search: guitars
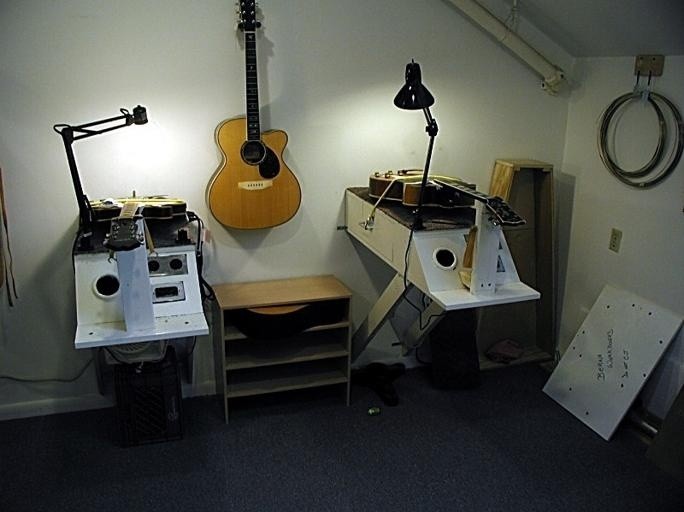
[369,169,526,227]
[87,194,187,250]
[206,0,301,230]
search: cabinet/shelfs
[211,274,352,425]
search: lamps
[394,59,439,230]
[62,105,148,235]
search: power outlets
[610,228,621,254]
